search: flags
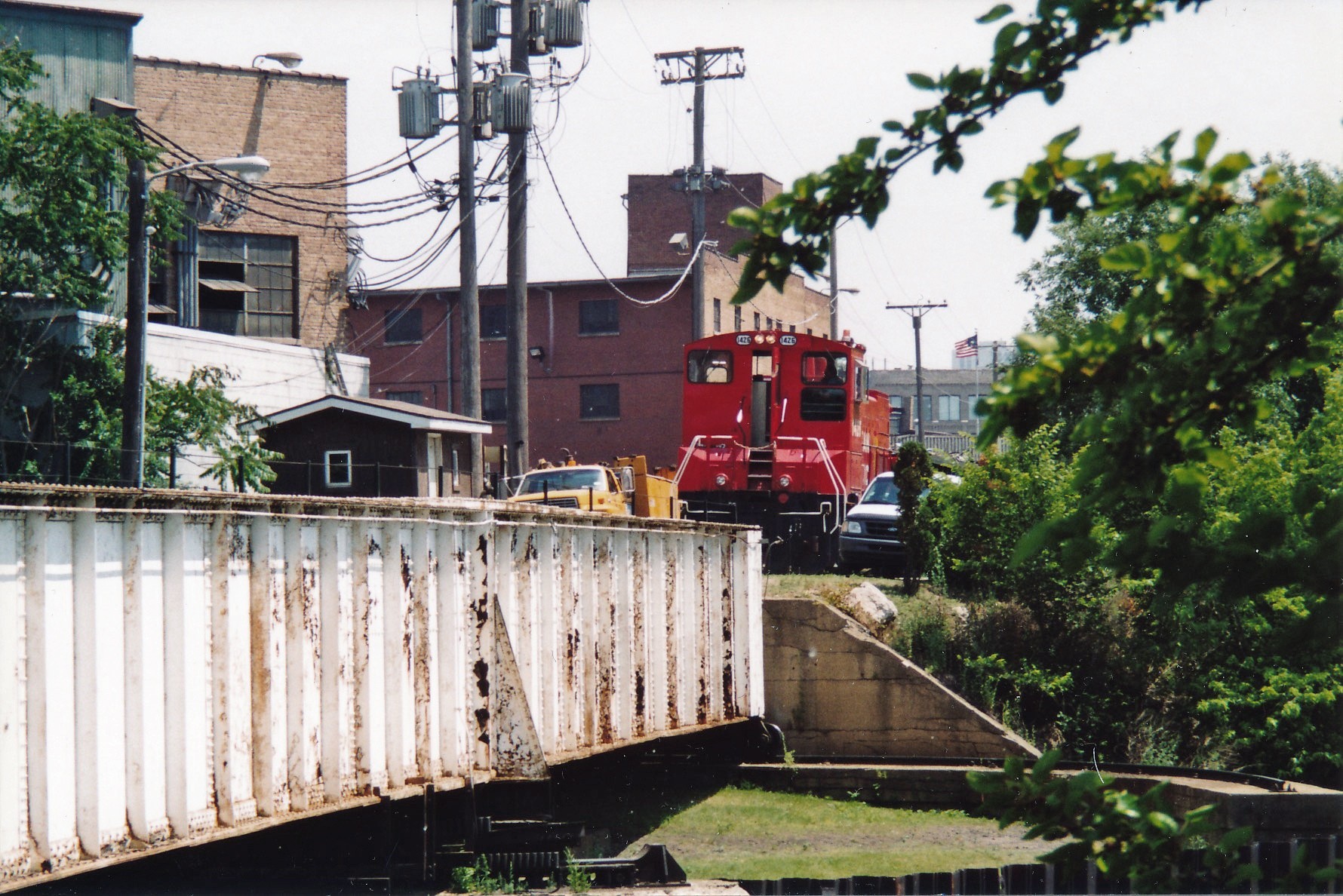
[955,334,977,357]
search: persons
[820,365,843,383]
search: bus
[674,325,900,566]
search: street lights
[120,147,269,491]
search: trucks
[507,447,682,519]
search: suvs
[839,471,967,571]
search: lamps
[528,346,544,360]
[253,51,303,73]
